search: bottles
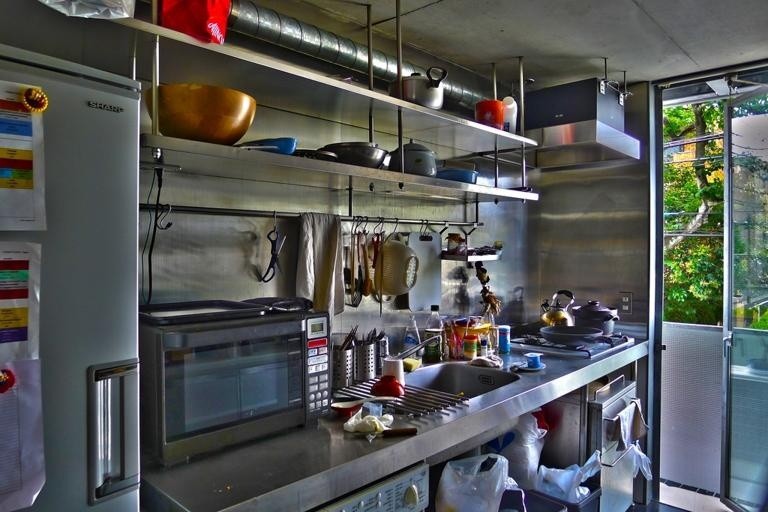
[501,97,518,134]
[480,340,487,355]
[498,325,511,354]
[446,232,466,256]
[424,304,445,358]
[482,311,495,328]
[402,315,421,358]
[462,335,478,361]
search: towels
[288,212,346,337]
[616,398,650,451]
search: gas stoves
[510,332,635,359]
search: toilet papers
[381,358,405,386]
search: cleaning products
[422,304,446,364]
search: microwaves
[145,309,332,468]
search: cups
[377,340,388,374]
[523,353,544,368]
[382,357,406,395]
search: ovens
[588,372,636,512]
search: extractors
[524,76,640,175]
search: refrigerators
[1,43,144,512]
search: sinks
[403,361,521,400]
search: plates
[514,362,546,370]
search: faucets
[390,336,446,361]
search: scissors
[259,230,288,283]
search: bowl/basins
[447,322,492,358]
[369,375,405,396]
[317,141,388,167]
[147,84,257,148]
[437,169,478,184]
[244,137,297,155]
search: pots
[540,325,612,348]
[572,299,618,335]
[389,65,448,110]
[387,138,437,178]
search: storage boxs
[532,469,602,512]
[525,491,568,512]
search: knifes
[353,427,417,438]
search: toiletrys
[401,315,423,365]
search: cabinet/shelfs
[0,0,539,204]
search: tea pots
[539,289,575,327]
